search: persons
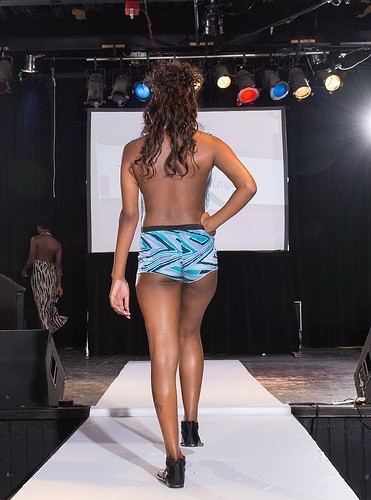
[20,216,70,334]
[106,62,259,489]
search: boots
[181,420,201,447]
[156,455,186,488]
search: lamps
[289,67,311,100]
[261,69,290,101]
[132,77,154,103]
[110,75,129,108]
[0,51,14,95]
[314,63,341,92]
[233,69,260,104]
[21,53,47,74]
[86,72,105,108]
[204,12,225,35]
[214,64,232,89]
[0,328,67,408]
[353,330,370,406]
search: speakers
[354,328,371,405]
[0,330,65,408]
